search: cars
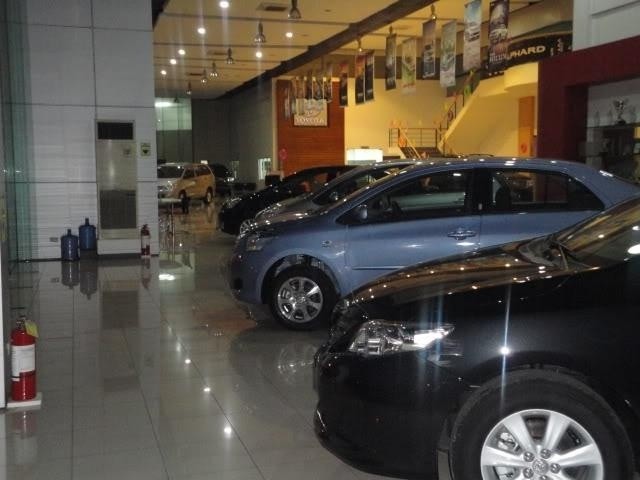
[402,19,508,76]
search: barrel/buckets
[60,262,80,290]
[257,157,272,181]
[60,229,80,262]
[80,253,99,301]
[79,217,98,251]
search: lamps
[172,0,301,103]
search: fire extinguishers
[10,315,39,401]
[140,223,151,256]
